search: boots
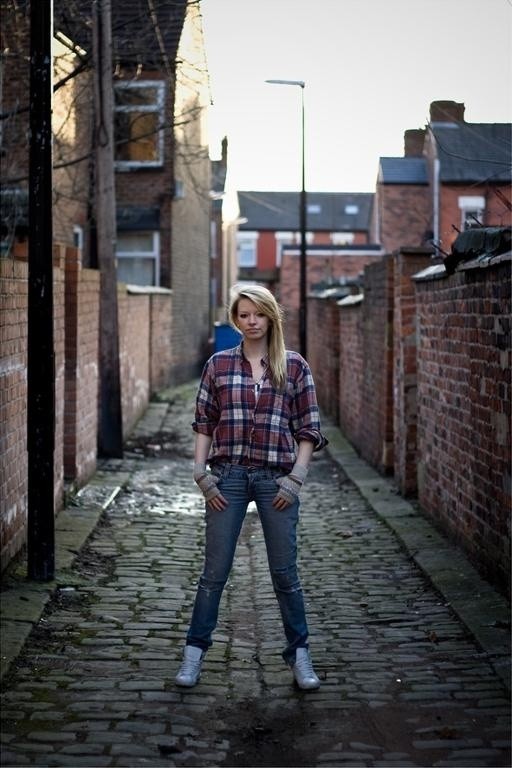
[174,646,205,687]
[293,648,321,691]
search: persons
[176,286,329,690]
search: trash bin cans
[214,321,243,353]
